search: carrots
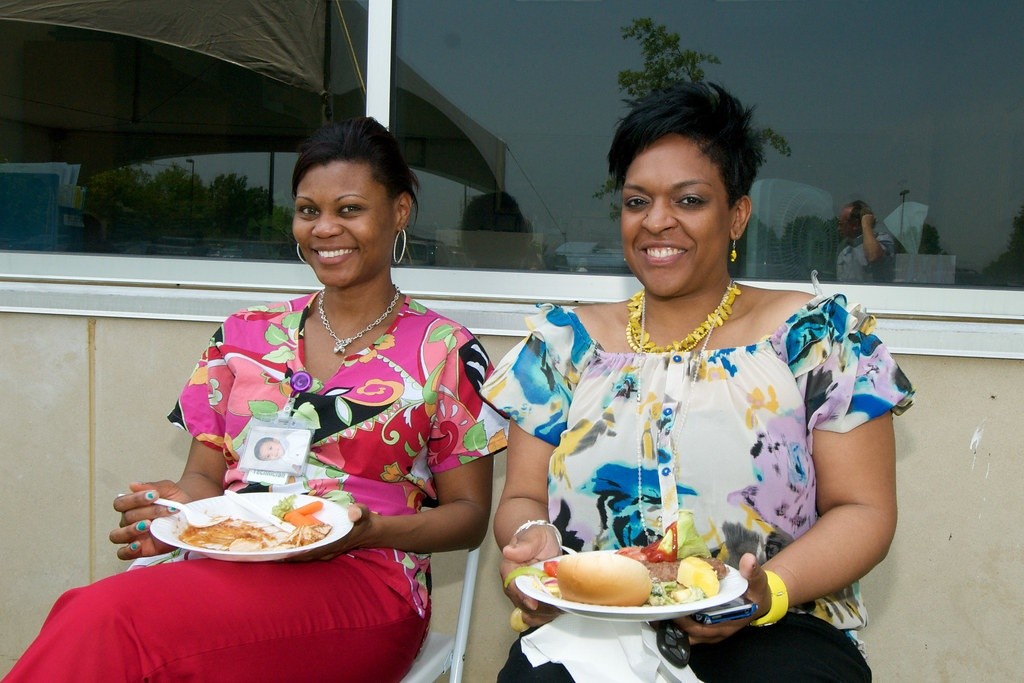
[283,501,323,528]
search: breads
[556,551,652,607]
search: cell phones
[690,596,758,626]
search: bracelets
[749,570,789,627]
[512,520,578,555]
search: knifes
[224,490,296,533]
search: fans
[745,178,841,281]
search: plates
[515,549,748,622]
[150,492,354,561]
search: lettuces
[653,509,712,560]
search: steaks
[636,555,725,582]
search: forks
[117,493,229,528]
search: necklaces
[314,286,401,356]
[626,280,742,542]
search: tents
[0,0,507,261]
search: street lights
[186,157,196,240]
[896,188,913,249]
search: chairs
[125,544,481,683]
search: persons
[0,117,507,683]
[481,81,916,683]
[835,201,897,284]
